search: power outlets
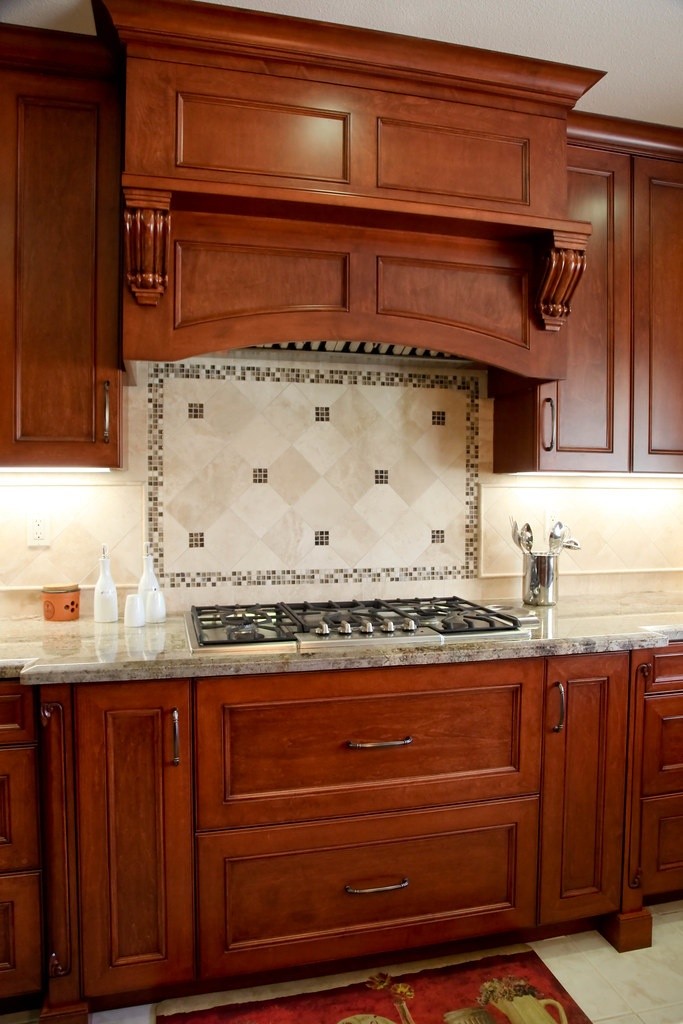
[28,513,49,547]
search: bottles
[138,542,160,597]
[93,543,118,622]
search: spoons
[549,521,564,555]
[520,523,533,554]
[512,521,525,554]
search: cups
[123,594,145,627]
[145,591,166,623]
[522,552,558,606]
[41,584,81,621]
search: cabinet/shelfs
[88,1,606,378]
[39,678,192,1024]
[196,652,547,982]
[540,646,631,959]
[0,681,37,1009]
[494,111,681,472]
[633,644,683,952]
[0,24,121,469]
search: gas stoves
[184,595,532,658]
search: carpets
[152,941,594,1024]
